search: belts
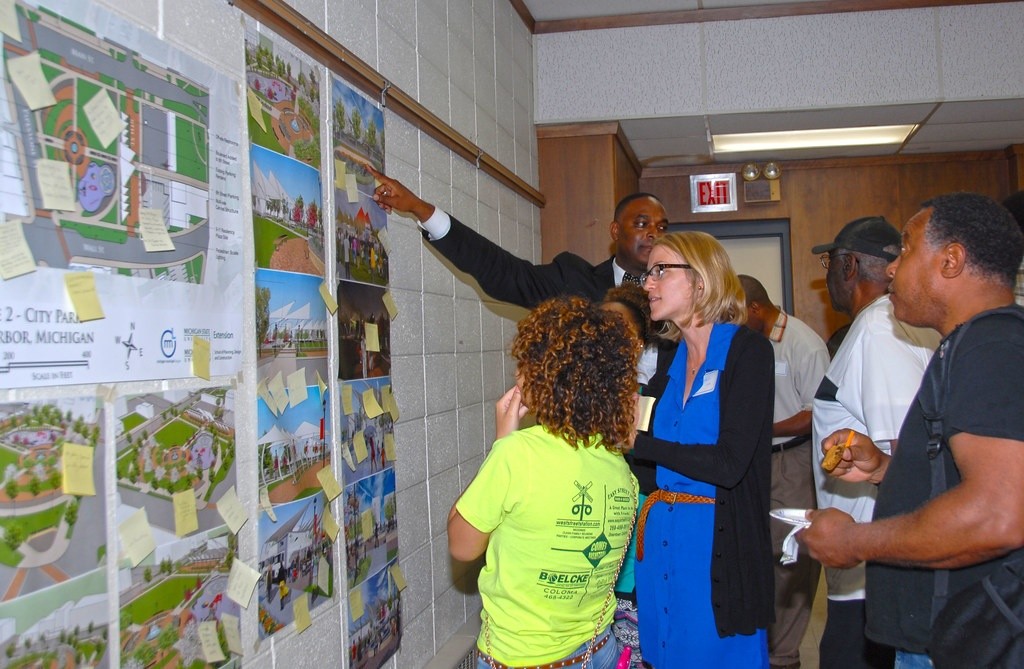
[636,489,716,561]
[771,432,812,453]
[474,633,612,669]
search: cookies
[822,446,843,471]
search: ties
[622,272,642,287]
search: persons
[365,163,671,310]
[737,274,831,669]
[447,285,663,668]
[274,576,288,610]
[369,436,386,474]
[342,242,389,283]
[811,214,943,669]
[624,231,775,669]
[348,635,363,669]
[374,522,379,548]
[795,193,1024,669]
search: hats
[810,215,903,263]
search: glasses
[638,263,692,284]
[819,251,861,269]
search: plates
[769,508,812,528]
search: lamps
[740,160,781,203]
